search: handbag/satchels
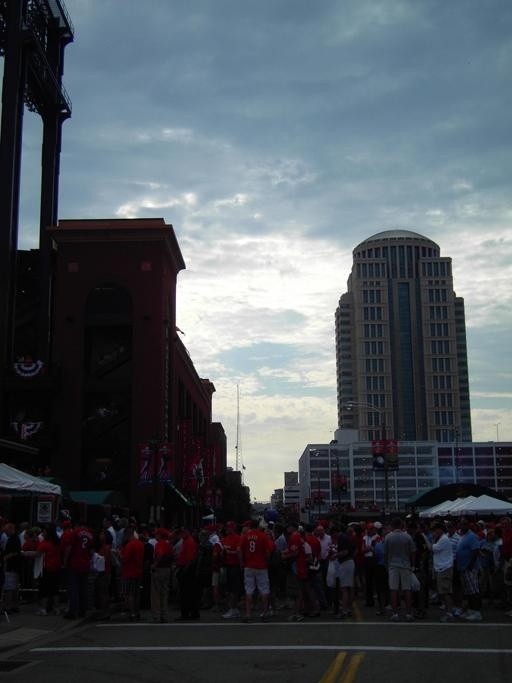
[267,551,284,576]
[158,555,172,568]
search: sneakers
[221,604,483,625]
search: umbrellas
[419,492,511,516]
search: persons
[1,512,512,626]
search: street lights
[342,397,393,514]
[327,439,343,506]
[308,448,322,518]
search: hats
[0,516,512,537]
